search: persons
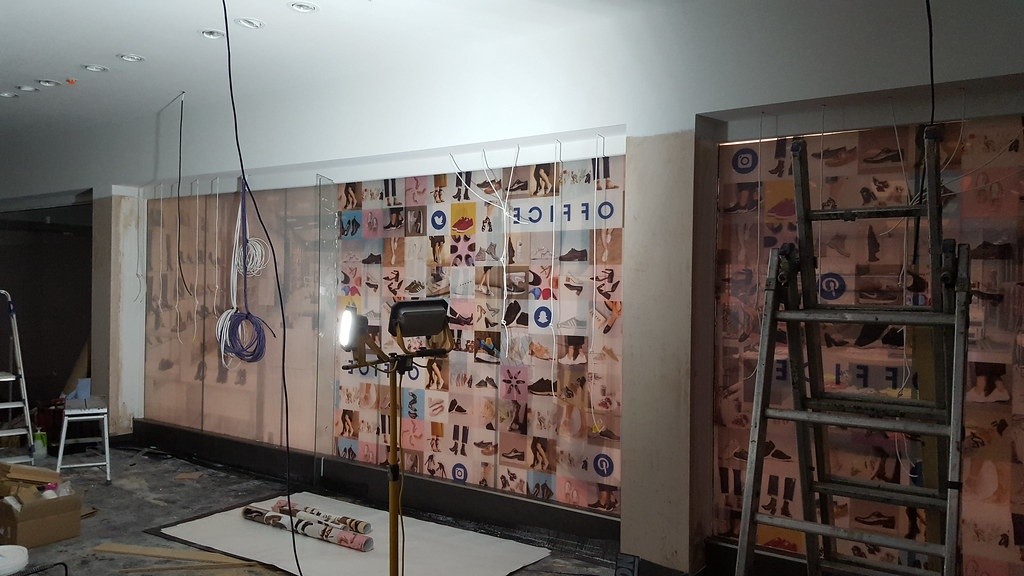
[533,437,549,470]
[383,179,403,206]
[433,174,447,203]
[449,425,469,457]
[343,183,358,210]
[425,455,448,481]
[601,228,614,263]
[558,336,588,365]
[592,156,619,190]
[342,409,354,437]
[716,464,796,521]
[534,164,552,196]
[478,266,495,297]
[427,359,444,390]
[587,483,620,512]
[389,238,402,265]
[603,301,622,334]
[431,236,445,264]
[453,172,472,202]
[383,207,405,231]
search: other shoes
[715,133,1024,572]
[333,169,620,516]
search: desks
[56,395,112,486]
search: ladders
[735,135,971,576]
[1,289,35,467]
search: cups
[78,378,90,400]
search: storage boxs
[0,493,81,548]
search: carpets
[141,488,552,576]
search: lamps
[387,299,449,338]
[338,303,368,353]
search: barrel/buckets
[28,432,48,459]
